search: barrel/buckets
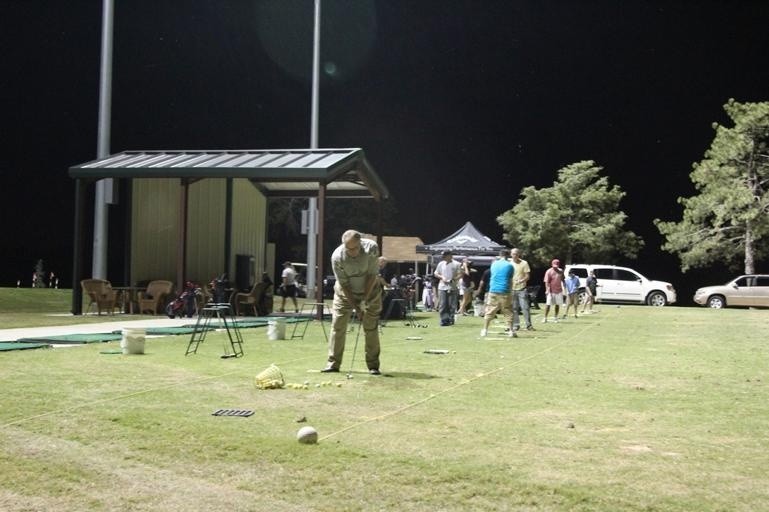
[267,320,285,340]
[122,328,145,355]
[211,280,232,303]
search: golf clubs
[213,306,236,358]
[550,292,560,323]
[346,303,365,376]
[400,301,429,328]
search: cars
[693,273,769,310]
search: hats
[552,259,560,268]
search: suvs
[560,263,676,305]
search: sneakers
[542,317,548,324]
[512,325,521,331]
[527,325,537,332]
[481,329,488,338]
[561,309,594,319]
[509,331,518,339]
[553,317,560,323]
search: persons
[319,229,383,375]
[541,259,598,323]
[280,261,302,313]
[376,256,422,310]
[422,248,536,336]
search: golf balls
[287,379,343,389]
[297,426,318,443]
[349,375,353,379]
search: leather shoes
[368,368,382,375]
[321,367,339,373]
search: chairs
[82,279,264,317]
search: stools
[383,299,417,328]
[184,303,328,358]
